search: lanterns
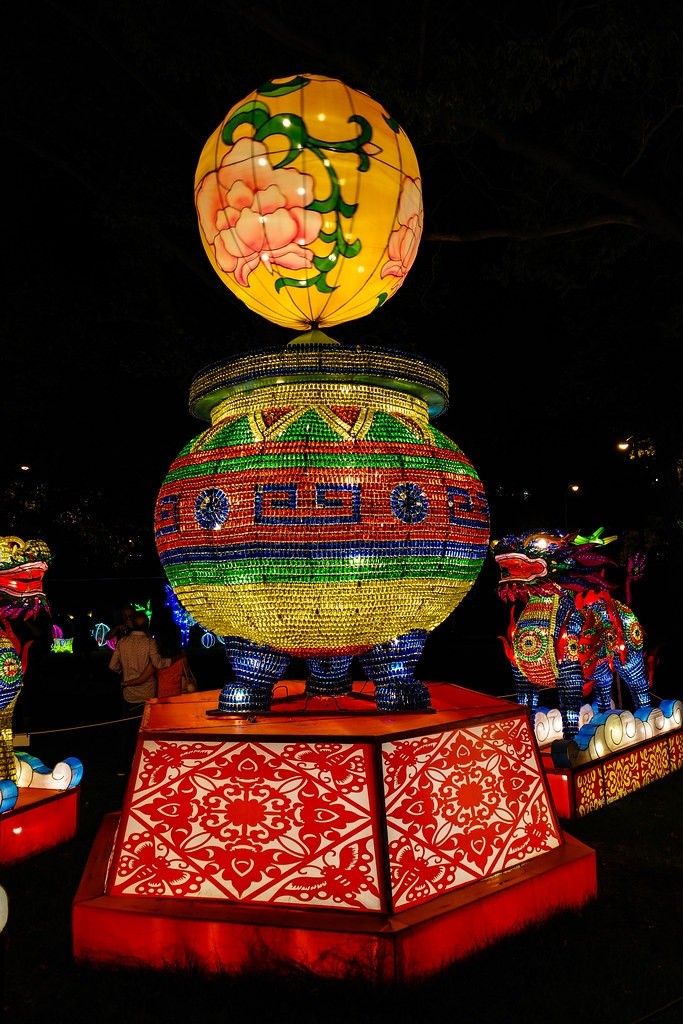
[193,73,424,327]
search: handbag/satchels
[181,659,199,693]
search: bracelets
[119,625,123,629]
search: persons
[105,606,194,777]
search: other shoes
[116,770,127,776]
